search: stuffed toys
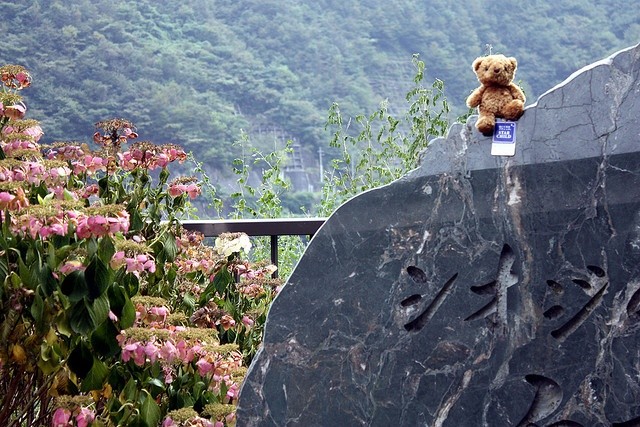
[466,55,526,134]
